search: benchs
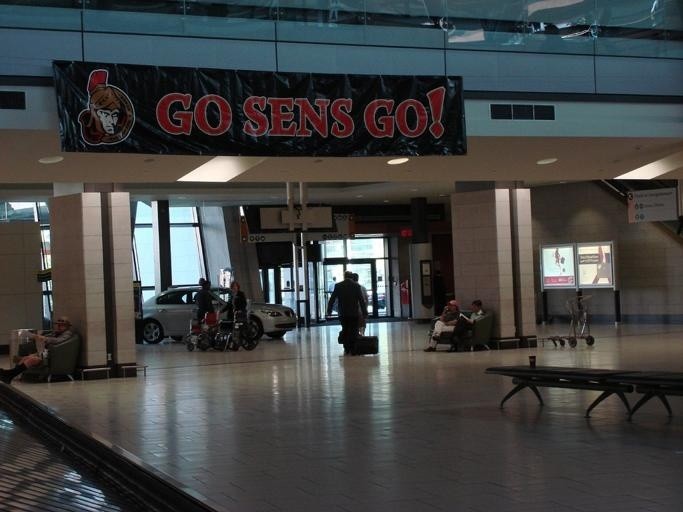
[428,309,493,352]
[483,364,683,425]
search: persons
[423,300,461,351]
[450,300,488,352]
[193,277,226,327]
[555,247,560,265]
[352,273,367,336]
[327,271,367,355]
[0,317,75,384]
[219,282,254,350]
[329,277,338,311]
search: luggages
[351,317,378,355]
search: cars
[142,284,296,344]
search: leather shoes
[423,346,436,352]
[433,336,440,341]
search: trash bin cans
[9,329,37,380]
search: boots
[0,364,22,385]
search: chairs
[18,330,81,382]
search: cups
[529,356,536,368]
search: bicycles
[223,317,260,351]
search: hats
[54,316,73,326]
[449,300,458,306]
